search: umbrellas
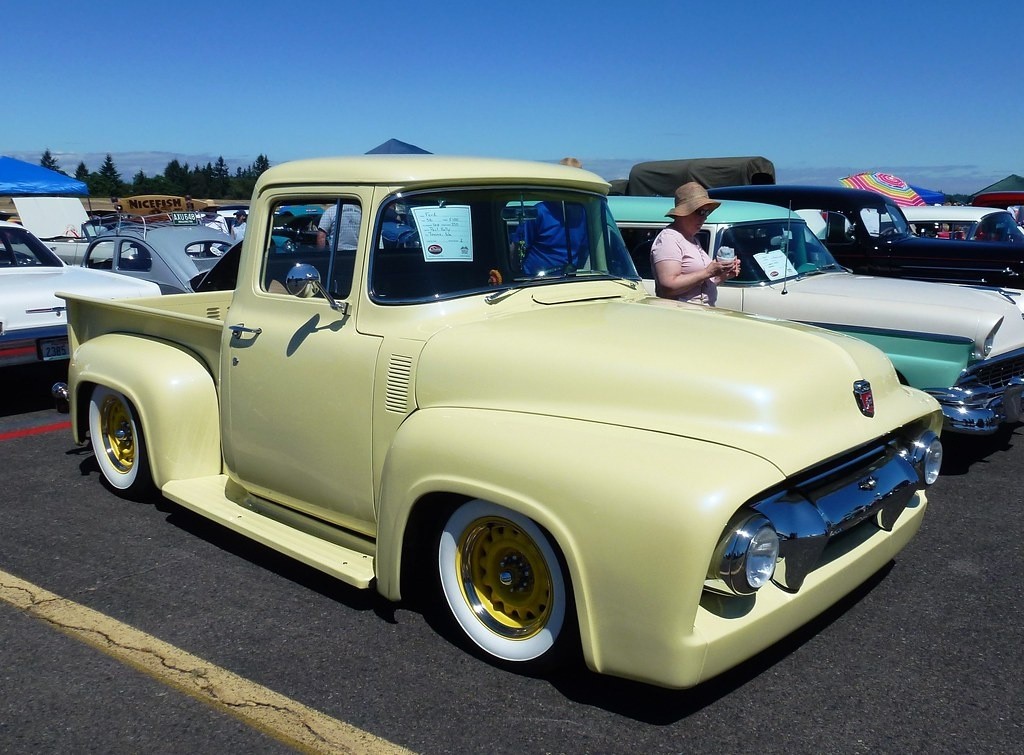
[837,172,928,208]
[0,155,91,198]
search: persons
[230,209,248,243]
[512,157,607,278]
[650,181,742,308]
[199,199,231,238]
[317,203,362,300]
[936,223,950,239]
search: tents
[908,183,946,205]
[967,174,1024,207]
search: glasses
[695,208,711,216]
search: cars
[12,199,326,295]
[505,195,1024,445]
[0,218,164,412]
[706,183,1024,289]
[47,150,945,695]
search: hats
[203,199,219,206]
[664,182,721,217]
[233,210,247,217]
